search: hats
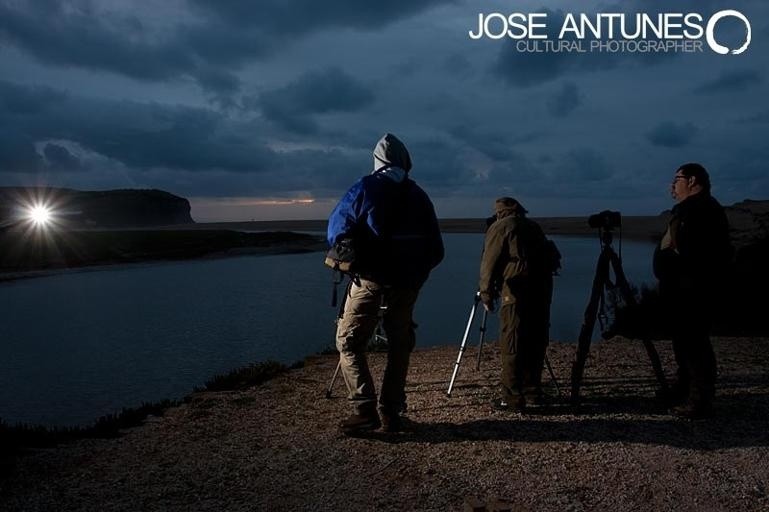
[373,132,412,172]
[496,197,523,212]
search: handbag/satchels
[325,230,356,273]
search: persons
[479,196,558,411]
[652,165,732,420]
[325,133,445,440]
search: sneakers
[339,412,381,430]
[490,394,545,411]
[382,412,414,432]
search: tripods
[570,244,668,404]
[444,278,563,403]
